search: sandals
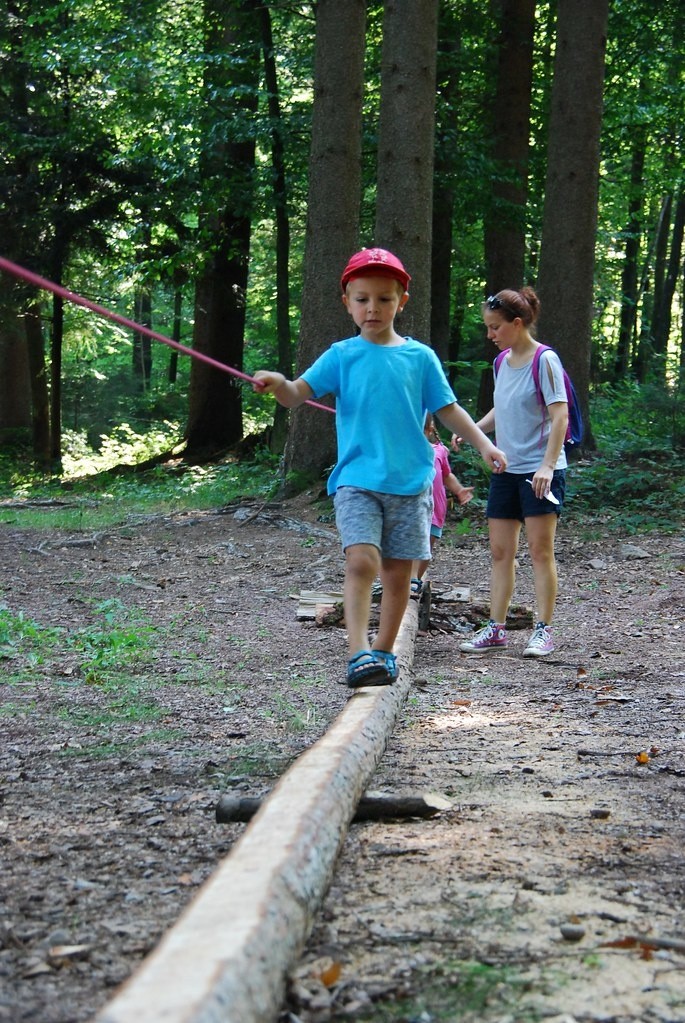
[410,578,423,599]
[371,650,397,682]
[347,650,388,688]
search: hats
[340,248,411,294]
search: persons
[409,411,475,598]
[451,286,569,657]
[252,248,508,688]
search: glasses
[487,296,524,318]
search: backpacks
[495,346,583,449]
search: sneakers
[459,619,508,653]
[523,622,555,657]
